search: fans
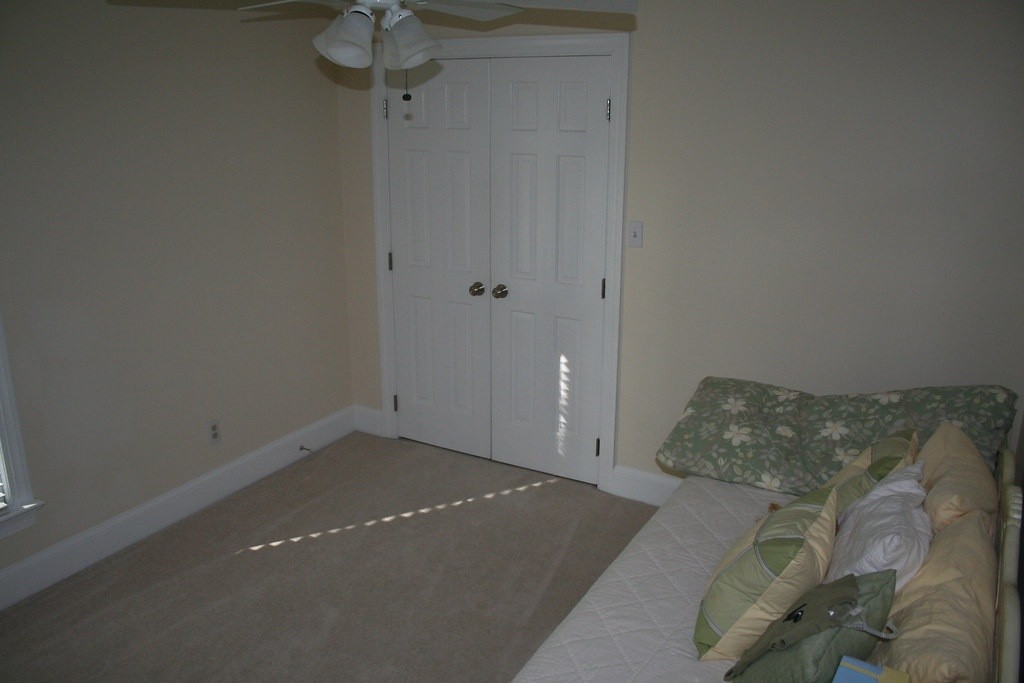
[238,0,638,23]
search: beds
[507,376,1024,683]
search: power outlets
[207,416,221,444]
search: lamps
[313,1,443,100]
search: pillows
[654,377,1018,683]
